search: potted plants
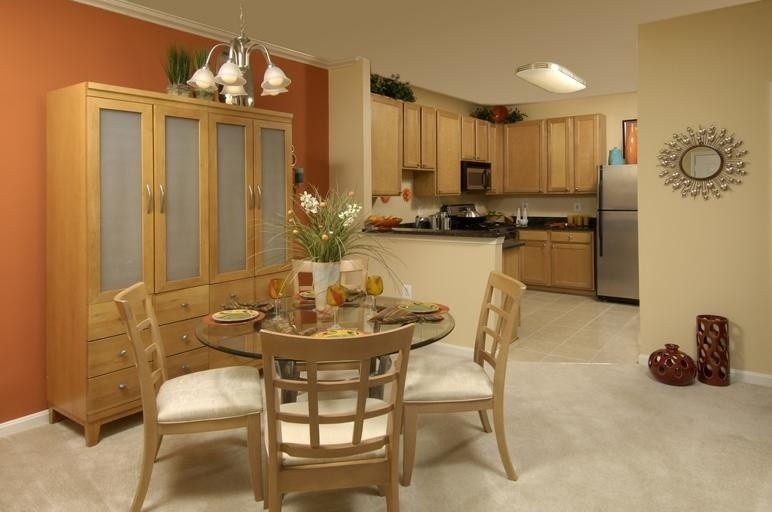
[193,45,213,99]
[159,41,191,97]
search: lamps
[516,60,587,94]
[186,1,291,97]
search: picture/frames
[622,119,638,158]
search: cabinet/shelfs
[518,230,597,296]
[370,92,607,197]
[207,100,295,370]
[44,81,210,448]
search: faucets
[418,214,438,230]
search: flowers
[245,161,411,304]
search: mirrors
[656,123,750,200]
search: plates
[298,293,314,299]
[398,302,440,314]
[212,309,259,323]
[367,216,402,226]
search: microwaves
[460,161,492,192]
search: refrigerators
[594,164,640,307]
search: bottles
[608,146,623,165]
[516,207,527,219]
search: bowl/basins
[518,219,528,226]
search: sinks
[391,226,438,232]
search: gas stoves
[450,220,516,231]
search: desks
[196,294,455,405]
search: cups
[567,215,589,226]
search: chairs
[258,323,419,512]
[113,281,265,512]
[292,259,371,296]
[389,269,526,484]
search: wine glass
[269,279,287,321]
[366,276,384,319]
[327,285,345,330]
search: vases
[648,343,697,386]
[608,146,625,165]
[696,314,731,387]
[313,260,342,318]
